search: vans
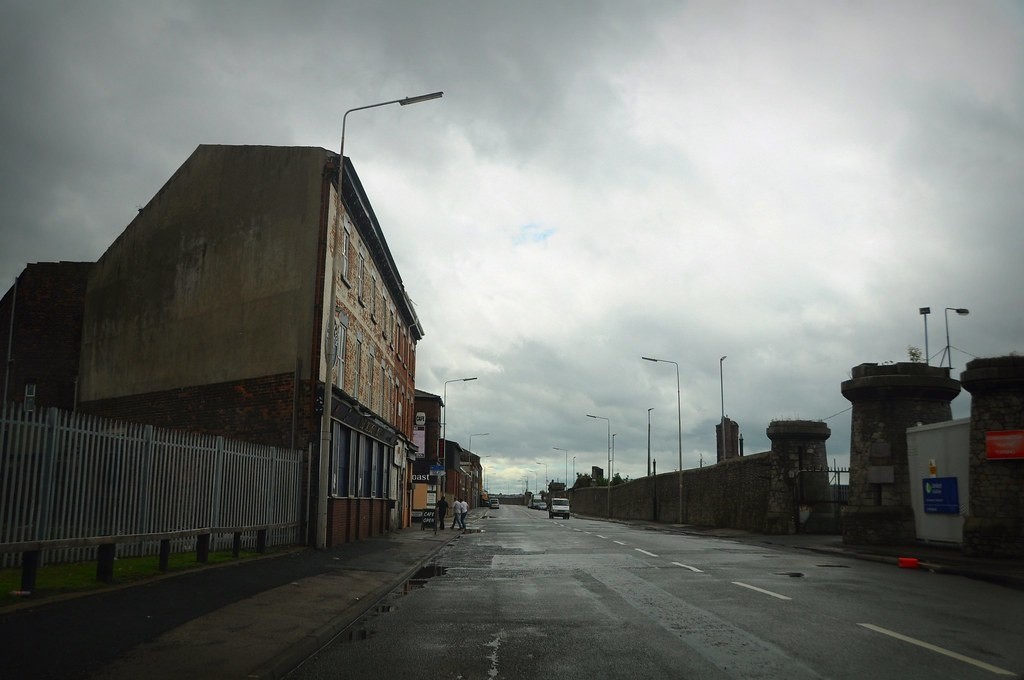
[548,498,570,520]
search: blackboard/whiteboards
[421,509,436,527]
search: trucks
[489,499,499,509]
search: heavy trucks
[528,493,542,509]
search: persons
[436,496,449,530]
[450,497,469,530]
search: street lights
[484,466,496,493]
[518,470,537,495]
[316,92,445,550]
[945,308,969,368]
[720,355,727,459]
[612,433,617,478]
[554,448,568,497]
[647,408,655,476]
[641,356,683,524]
[442,377,478,496]
[587,415,610,518]
[537,463,547,495]
[920,307,931,364]
[468,433,490,470]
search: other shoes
[458,528,463,530]
[450,527,453,529]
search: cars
[538,503,548,511]
